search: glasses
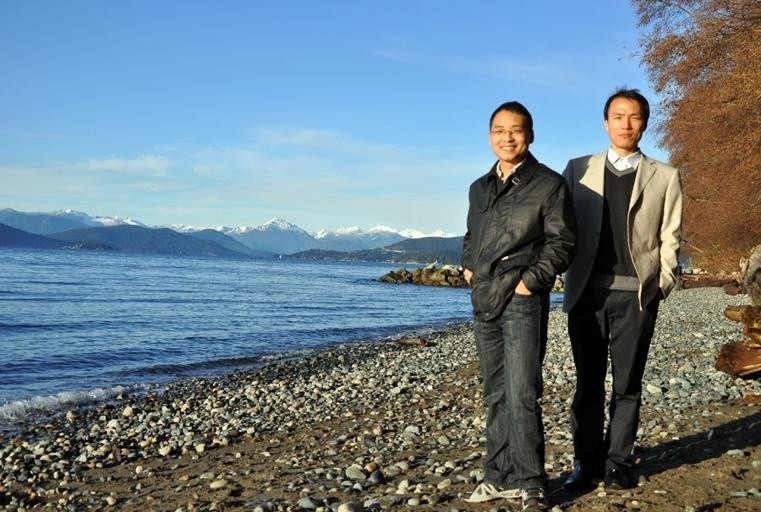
[490,127,529,137]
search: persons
[459,100,581,512]
[545,88,688,506]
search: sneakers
[521,487,546,512]
[604,458,624,484]
[458,479,522,503]
[564,464,595,490]
